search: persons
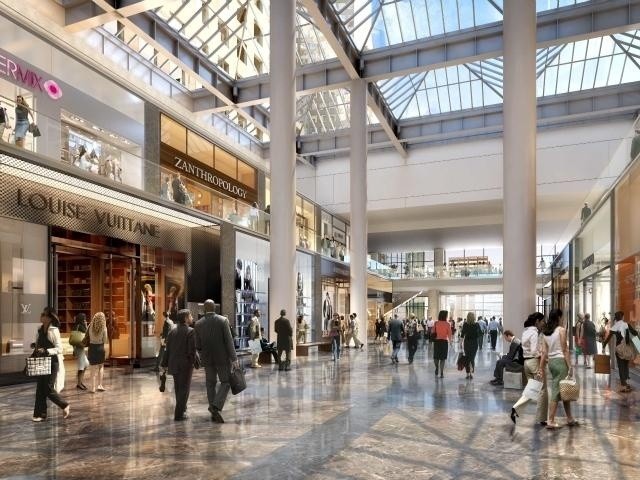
[235,260,242,289]
[72,148,94,170]
[244,267,253,291]
[172,173,188,203]
[72,312,88,391]
[141,283,154,321]
[31,306,70,422]
[85,312,105,389]
[264,204,271,234]
[405,263,409,275]
[538,259,548,273]
[249,309,297,371]
[14,95,35,148]
[297,314,307,344]
[321,234,343,258]
[195,299,241,424]
[162,281,183,340]
[328,310,363,361]
[160,308,195,421]
[251,200,258,229]
[163,177,170,199]
[0,102,9,141]
[373,311,640,430]
[580,202,592,223]
[414,260,502,277]
[323,292,331,332]
[631,128,640,156]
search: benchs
[296,341,334,357]
[503,366,523,390]
[236,348,274,369]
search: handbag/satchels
[69,330,89,347]
[616,341,636,360]
[459,356,466,370]
[26,357,51,376]
[229,369,246,395]
[246,325,259,337]
[559,379,579,401]
[594,354,610,373]
[429,332,437,344]
[523,379,543,401]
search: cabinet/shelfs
[58,257,130,335]
